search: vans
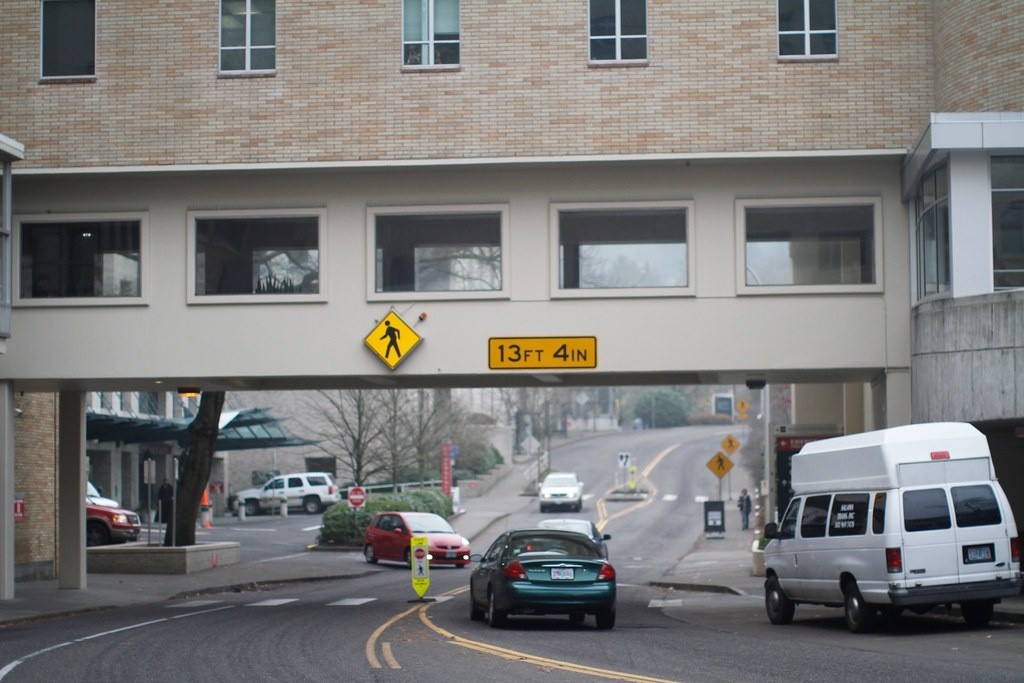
[762,421,1022,635]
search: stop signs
[415,548,425,560]
[349,487,366,506]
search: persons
[737,489,752,531]
[159,479,174,523]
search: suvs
[230,472,342,517]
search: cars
[86,505,142,548]
[538,518,611,562]
[363,511,471,569]
[86,482,119,510]
[469,528,616,630]
[537,472,584,513]
